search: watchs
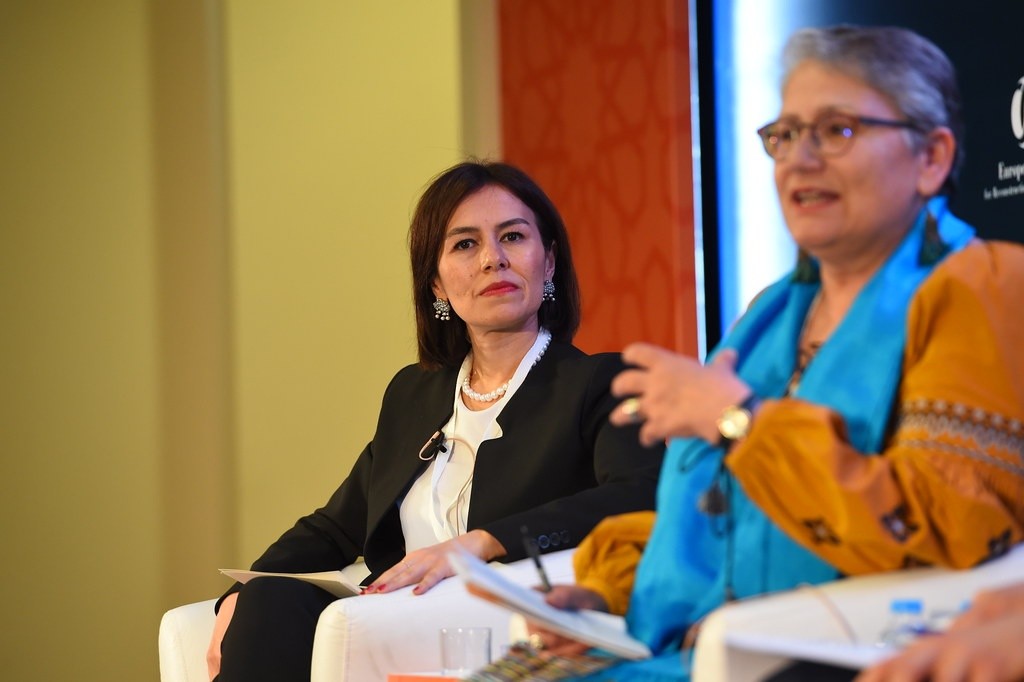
[715,392,765,452]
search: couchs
[159,547,579,682]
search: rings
[624,399,639,415]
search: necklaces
[461,333,551,403]
[800,296,823,342]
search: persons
[208,162,669,682]
[468,20,1024,682]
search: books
[452,547,655,667]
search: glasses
[757,113,927,161]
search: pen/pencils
[520,521,553,594]
